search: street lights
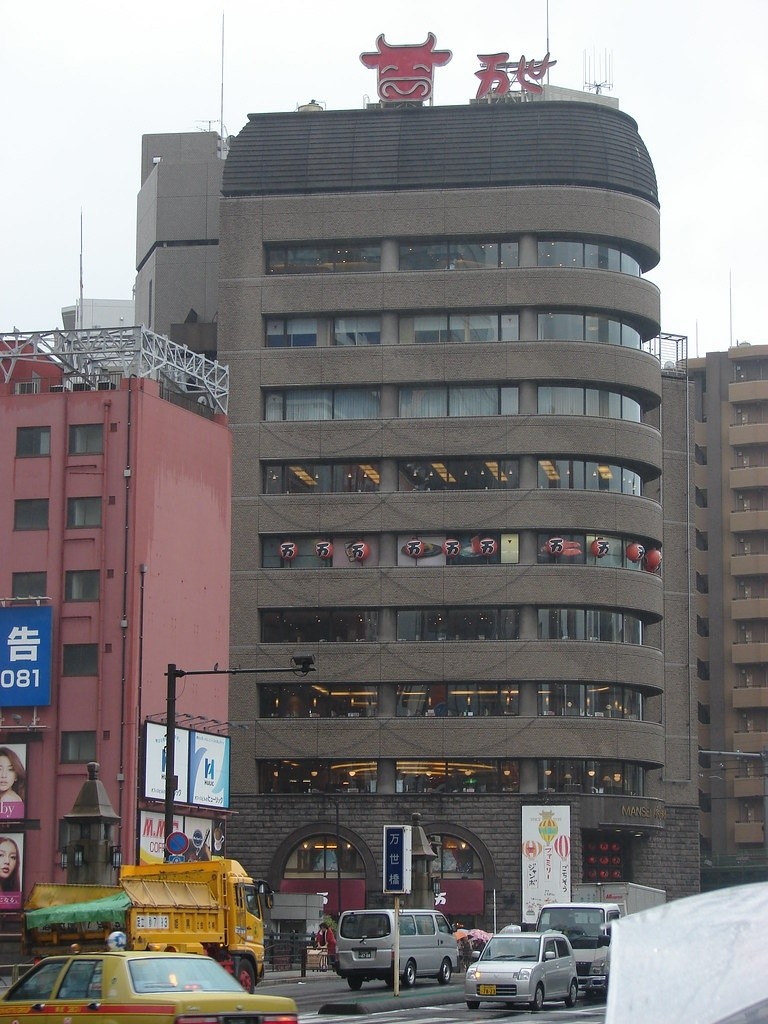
[311,788,341,913]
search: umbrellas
[454,929,489,940]
[499,923,521,933]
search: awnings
[434,879,485,916]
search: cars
[464,934,580,1011]
[0,932,299,1024]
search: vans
[334,909,460,990]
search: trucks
[522,881,667,994]
[22,858,273,994]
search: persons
[318,923,337,971]
[495,939,516,954]
[458,937,488,969]
[0,837,21,892]
[0,747,25,818]
